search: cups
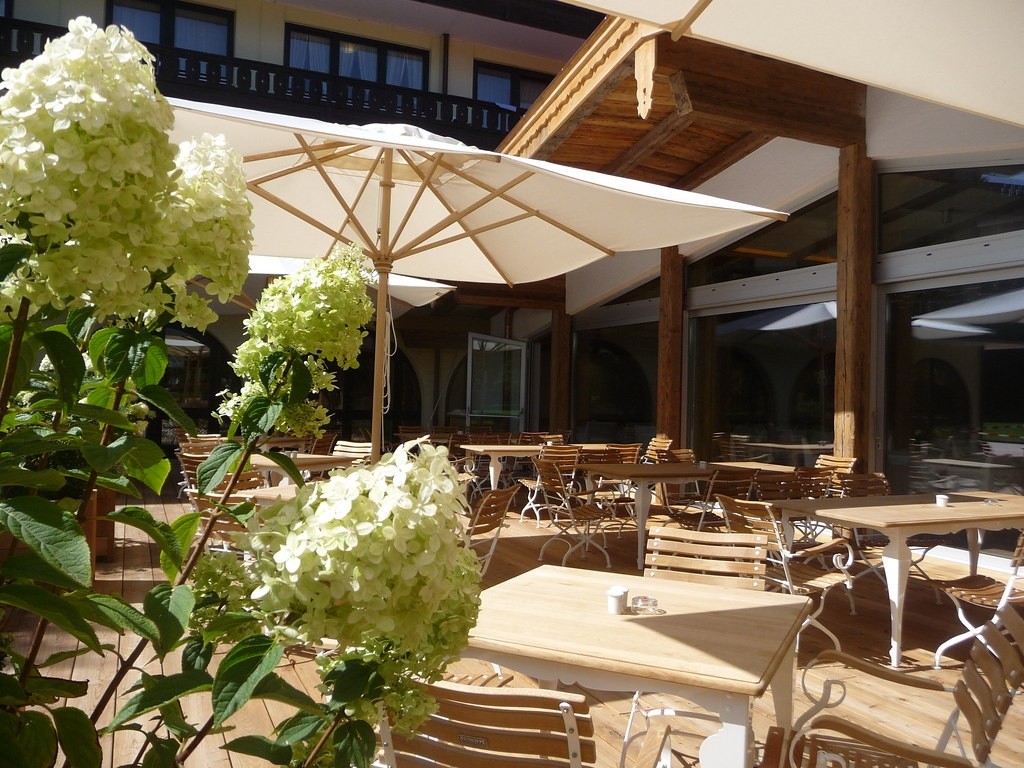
[699,461,707,469]
[936,495,949,508]
[292,450,298,459]
[547,441,553,446]
[286,451,291,458]
[606,591,624,615]
[611,586,629,610]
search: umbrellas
[162,335,209,397]
[2,66,791,467]
[185,254,455,315]
[716,298,993,441]
[911,285,1024,327]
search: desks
[582,462,798,571]
[450,564,813,767]
[748,440,834,466]
[245,479,323,506]
[227,452,356,488]
[920,456,1014,488]
[459,444,610,518]
[770,488,1024,666]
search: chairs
[176,425,1024,768]
